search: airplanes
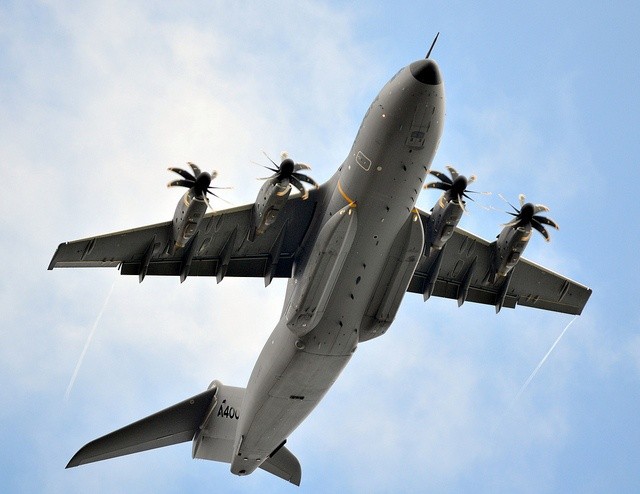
[47,30,594,487]
